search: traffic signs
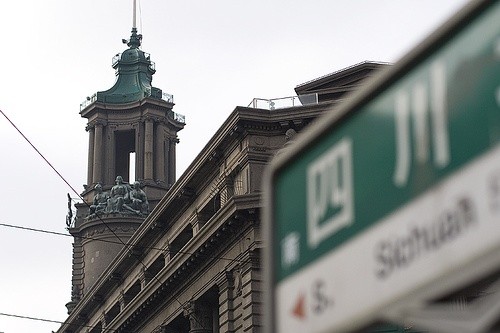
[262,0,500,332]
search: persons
[107,176,129,213]
[130,181,149,214]
[90,183,108,214]
